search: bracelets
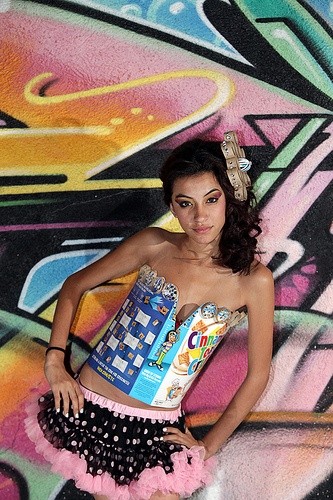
[45,346,66,357]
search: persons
[22,122,273,500]
